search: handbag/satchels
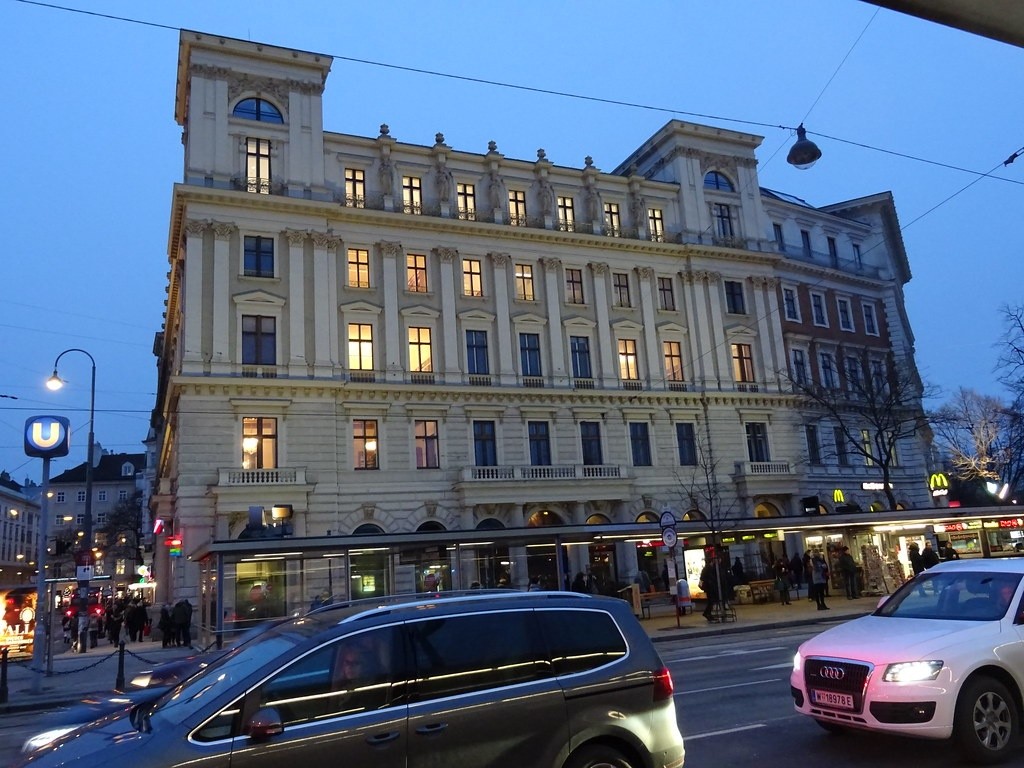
[698,581,704,590]
[144,625,150,636]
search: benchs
[750,579,799,603]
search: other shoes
[703,612,714,621]
[183,644,191,646]
[139,640,144,642]
[809,598,812,602]
[847,594,859,600]
[823,593,832,597]
[162,643,182,648]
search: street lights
[45,347,97,595]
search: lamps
[787,123,822,171]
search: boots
[64,637,70,644]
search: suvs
[3,588,687,768]
[790,555,1023,763]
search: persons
[909,541,960,576]
[999,582,1024,625]
[572,570,618,598]
[423,573,441,598]
[772,546,858,610]
[467,581,481,595]
[54,590,193,653]
[496,578,507,590]
[636,564,669,594]
[307,591,334,613]
[700,556,747,621]
[327,644,387,713]
[528,573,555,592]
